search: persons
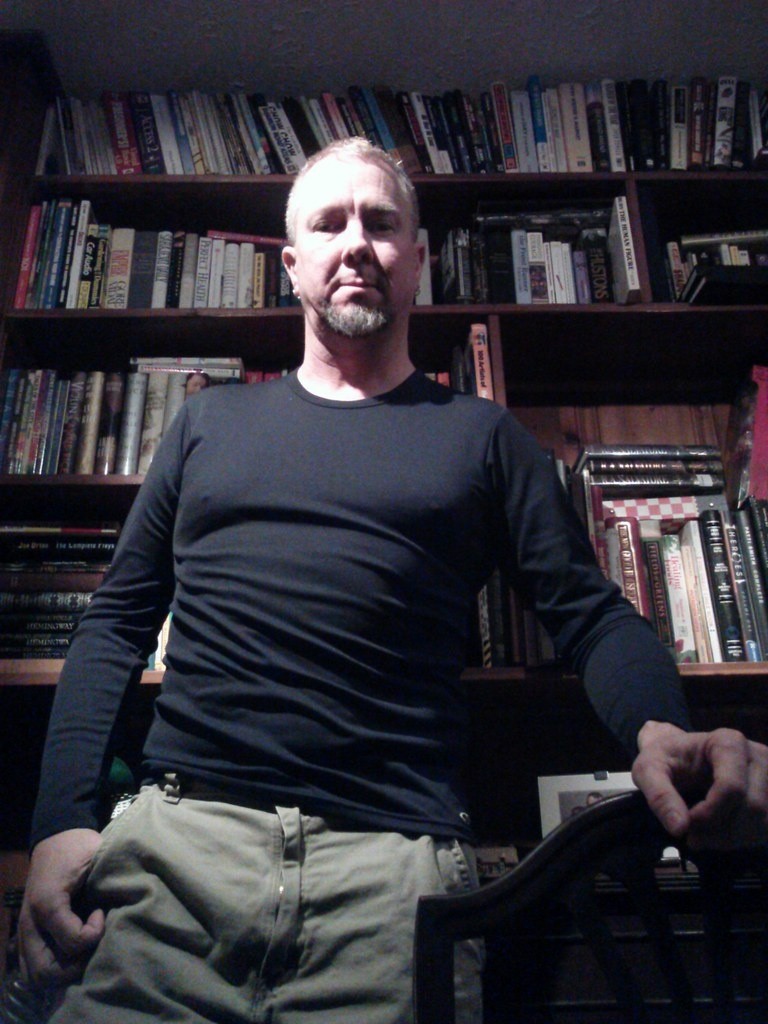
[16,135,767,1023]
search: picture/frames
[536,770,682,866]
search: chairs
[413,780,768,1023]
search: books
[0,441,767,664]
[10,195,768,312]
[0,320,489,472]
[37,74,765,172]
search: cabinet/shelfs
[1,82,768,684]
